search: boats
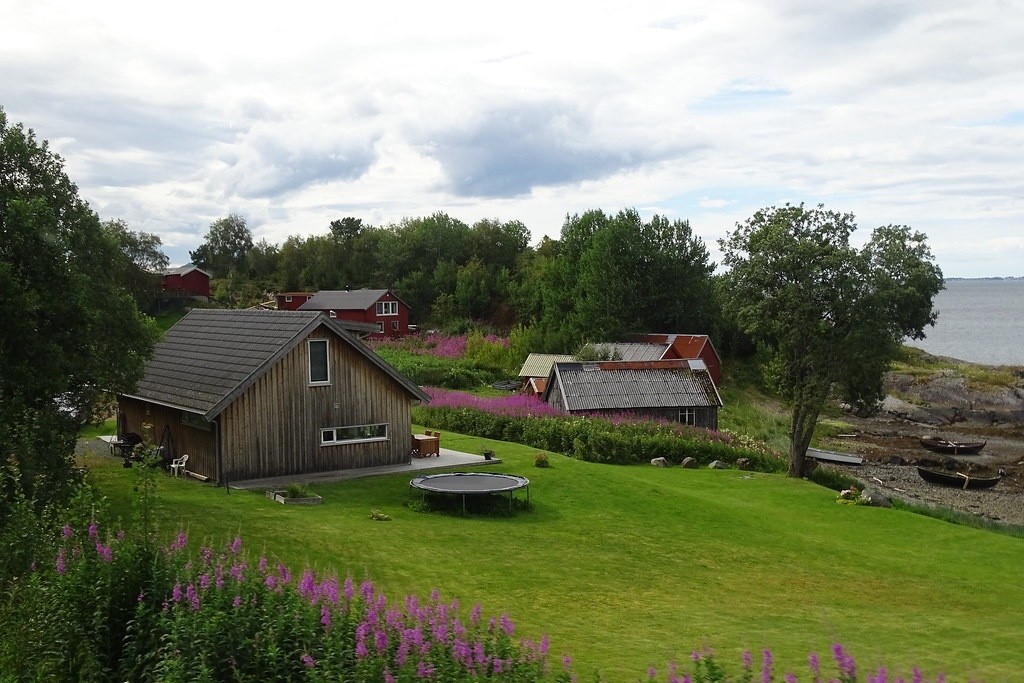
[919,436,987,455]
[915,467,1006,490]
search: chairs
[411,430,441,457]
[169,454,189,477]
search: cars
[53,390,93,428]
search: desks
[414,434,439,458]
